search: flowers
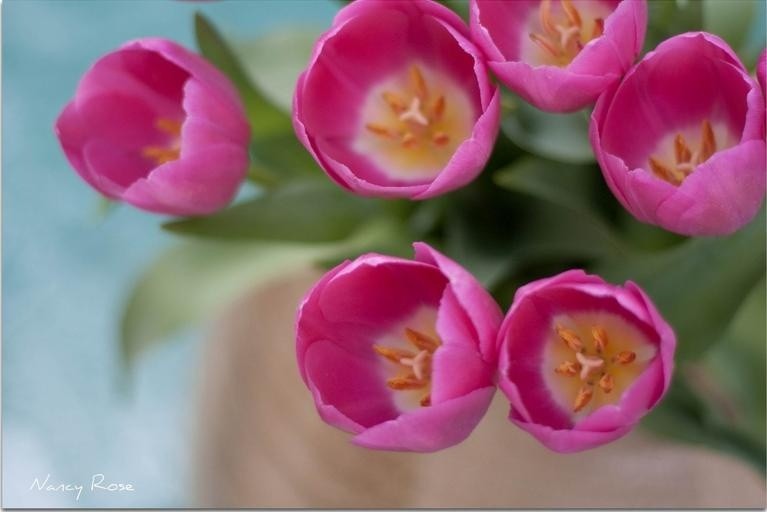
[52,0,765,452]
